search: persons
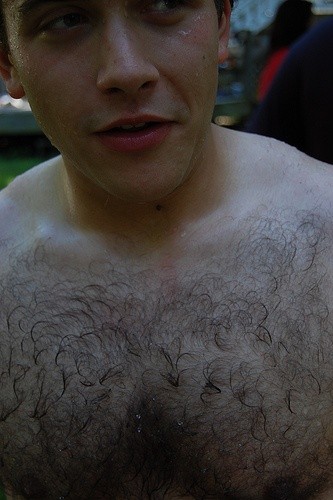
[0,1,332,498]
